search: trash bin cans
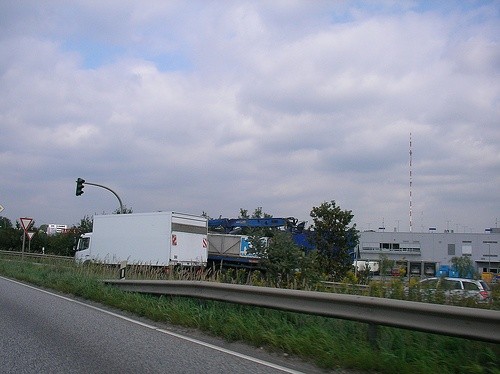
[481,272,494,280]
[448,265,461,278]
[460,266,475,279]
[435,265,449,277]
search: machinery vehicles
[205,216,306,281]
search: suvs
[385,276,494,306]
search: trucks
[72,211,208,274]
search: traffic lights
[76,178,85,196]
